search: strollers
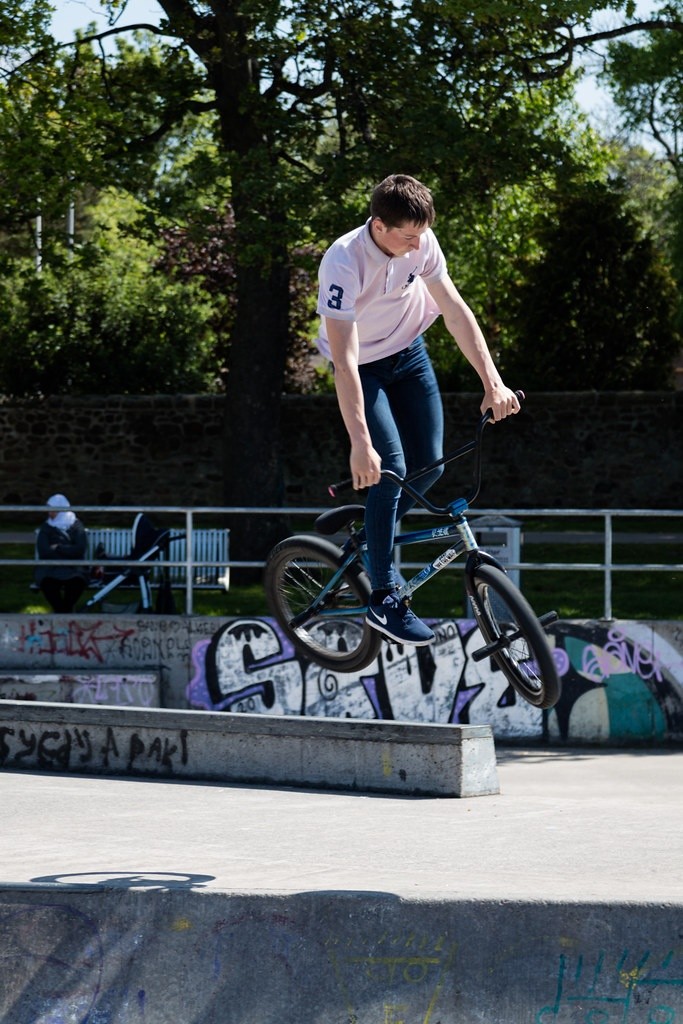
[86,514,187,621]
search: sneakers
[340,538,413,601]
[365,593,436,646]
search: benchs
[28,526,229,615]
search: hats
[47,494,71,509]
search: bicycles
[261,389,564,710]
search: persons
[311,174,522,645]
[32,494,89,613]
